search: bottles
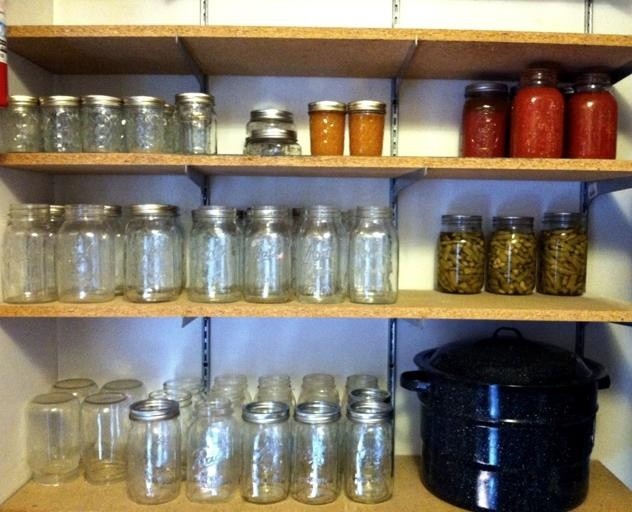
[436,210,587,297]
[126,374,394,505]
[243,107,301,155]
[122,95,176,152]
[0,201,401,308]
[171,93,218,155]
[461,67,618,161]
[26,377,146,488]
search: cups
[347,101,386,157]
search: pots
[398,325,612,511]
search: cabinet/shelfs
[1,21,632,512]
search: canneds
[104,205,124,293]
[460,82,506,159]
[80,394,128,480]
[247,108,297,137]
[433,215,487,295]
[251,384,293,406]
[210,387,246,423]
[52,202,116,301]
[25,393,83,486]
[565,75,616,160]
[187,207,240,304]
[149,390,190,462]
[52,378,94,392]
[344,400,394,501]
[184,398,240,501]
[243,128,301,156]
[240,401,289,499]
[484,216,537,290]
[540,211,586,293]
[290,402,345,505]
[257,375,292,390]
[0,202,55,303]
[348,203,399,300]
[118,96,164,153]
[343,98,384,154]
[162,102,175,152]
[165,376,204,409]
[300,374,334,395]
[237,203,292,303]
[213,375,252,406]
[347,389,390,407]
[174,92,217,151]
[102,377,148,401]
[343,373,379,409]
[295,204,347,304]
[300,386,340,406]
[127,398,182,497]
[120,201,185,304]
[509,69,564,158]
